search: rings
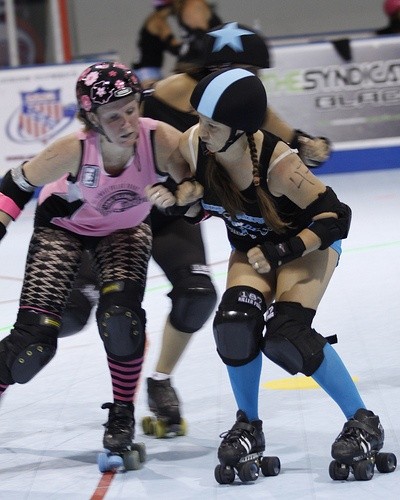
[155,192,160,197]
[254,262,260,268]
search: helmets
[190,68,268,131]
[194,21,271,68]
[76,60,145,119]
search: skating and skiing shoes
[328,408,398,482]
[214,410,281,485]
[142,376,187,439]
[97,401,147,473]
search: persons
[59,0,400,485]
[0,60,206,473]
